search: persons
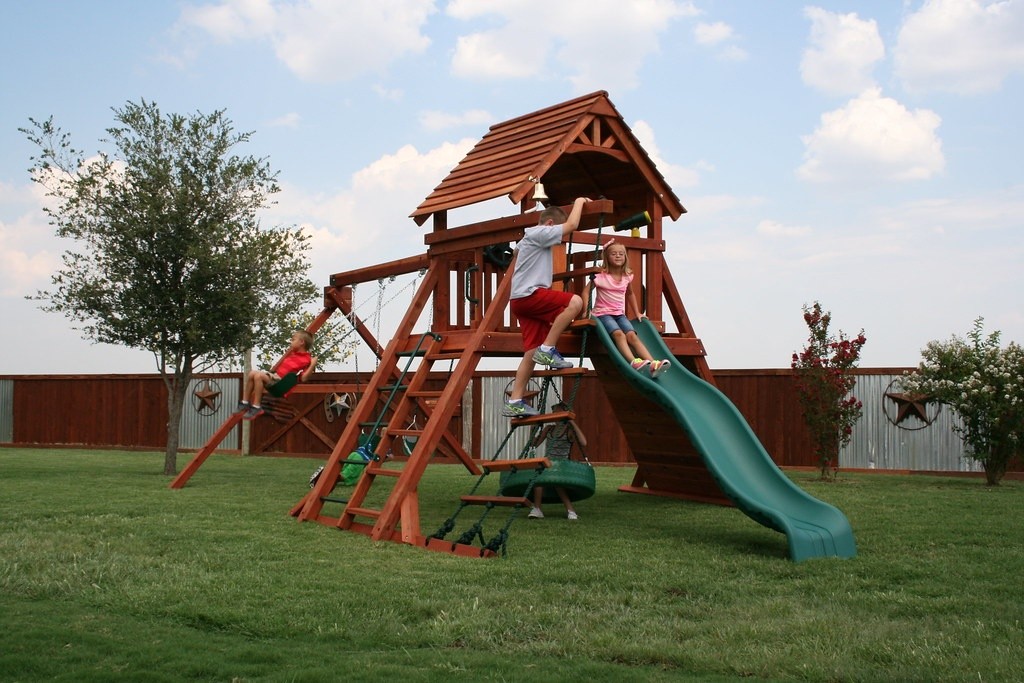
[527,401,588,520]
[308,426,396,490]
[231,327,319,421]
[581,237,672,379]
[500,196,595,417]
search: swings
[263,271,423,400]
[500,357,596,507]
[349,280,386,464]
[402,269,470,456]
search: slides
[591,317,863,562]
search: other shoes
[528,507,544,518]
[567,511,578,520]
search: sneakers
[501,400,540,417]
[233,400,251,417]
[630,358,651,373]
[243,407,264,420]
[531,345,573,368]
[650,359,671,378]
